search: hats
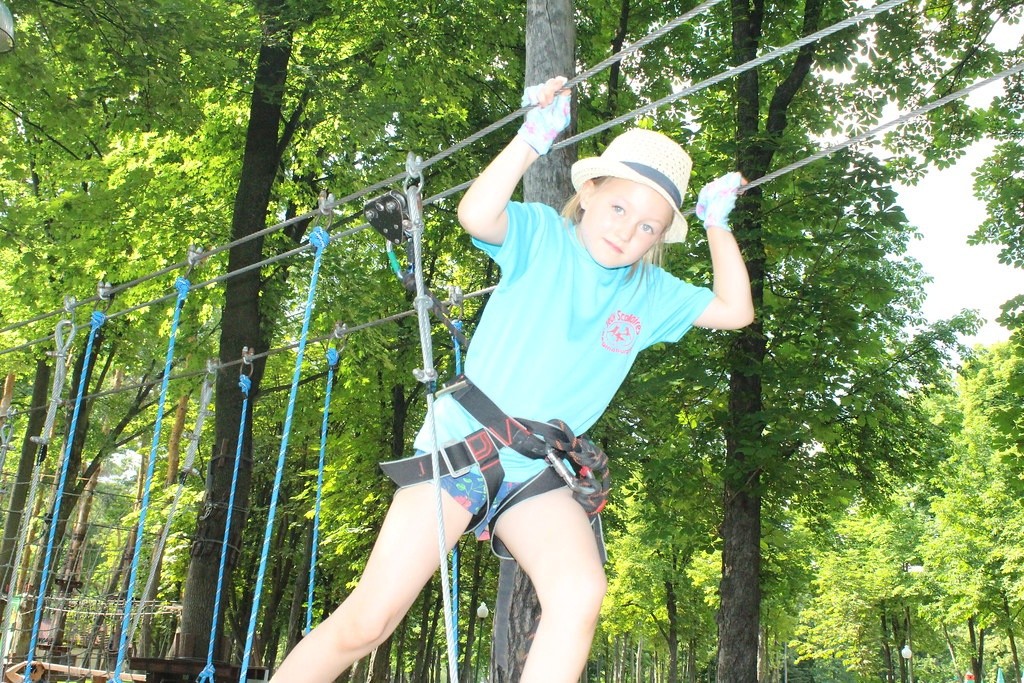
[570,128,693,244]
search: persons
[268,76,754,683]
